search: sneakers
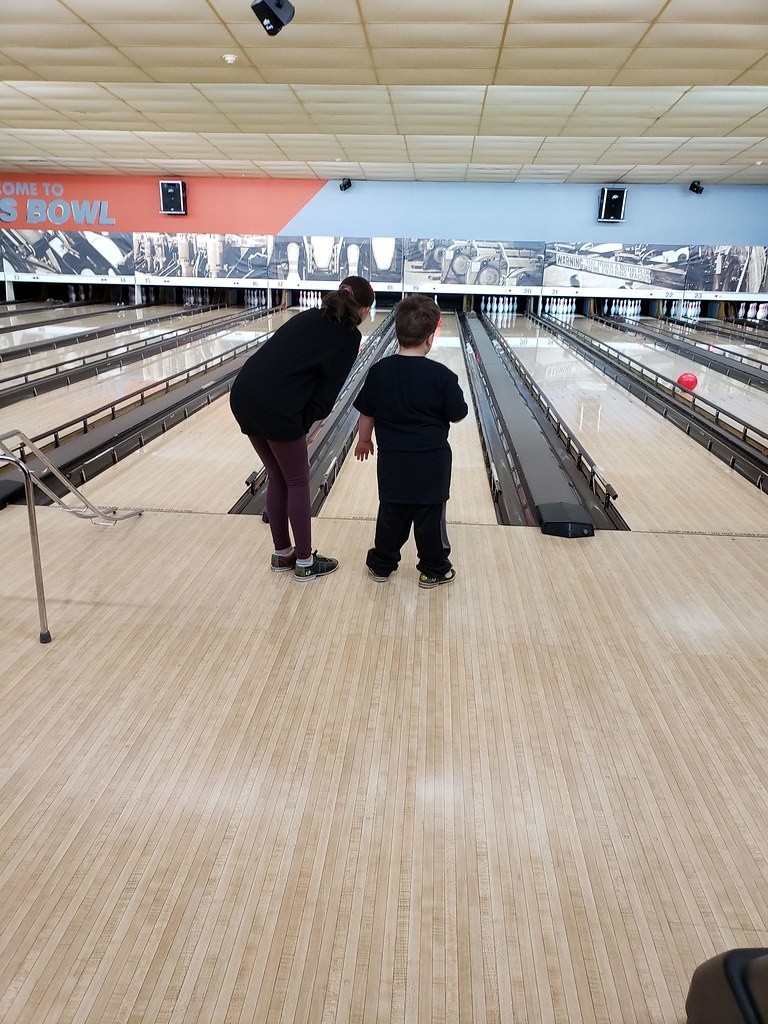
[293,549,339,582]
[270,546,297,572]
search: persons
[230,275,374,581]
[352,295,469,588]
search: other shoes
[418,568,457,589]
[367,567,389,582]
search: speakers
[251,0,295,36]
[597,187,628,222]
[160,181,187,212]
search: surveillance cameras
[689,183,704,194]
[340,180,351,191]
